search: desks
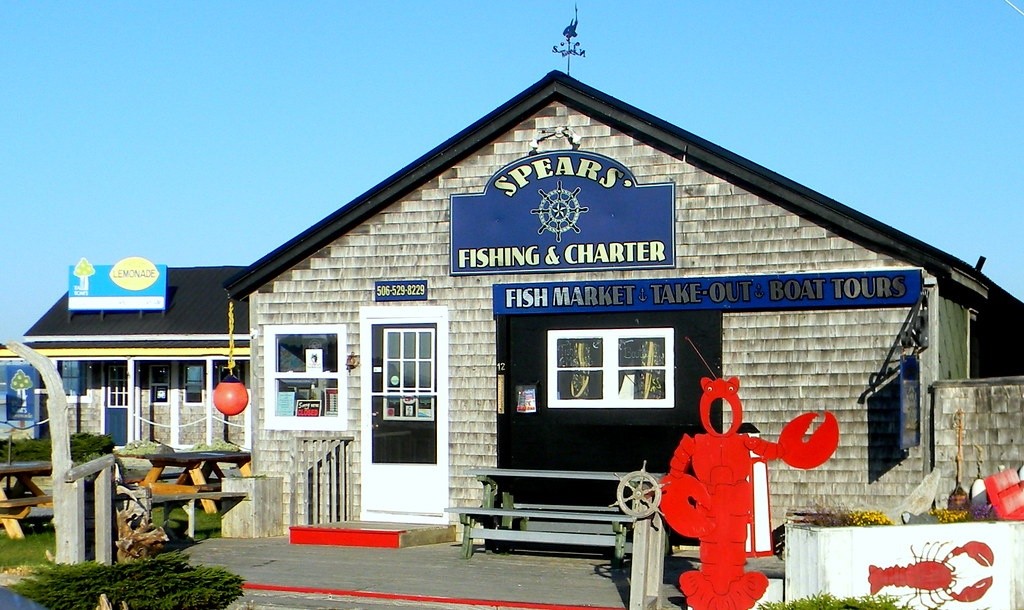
[0,461,53,539]
[462,467,673,556]
[137,451,251,514]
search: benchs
[0,495,53,540]
[124,472,183,488]
[443,503,636,569]
[194,482,221,514]
